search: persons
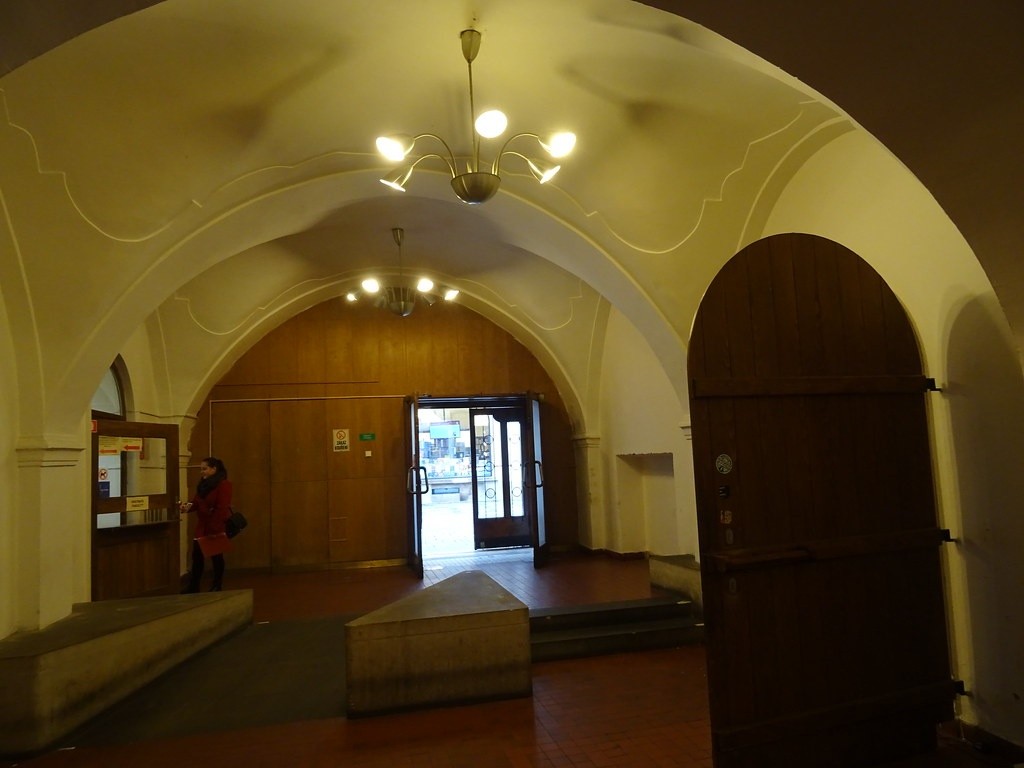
[180,457,232,595]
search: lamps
[344,226,461,317]
[373,29,579,205]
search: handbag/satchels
[225,513,248,539]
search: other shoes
[208,584,222,592]
[183,586,200,593]
